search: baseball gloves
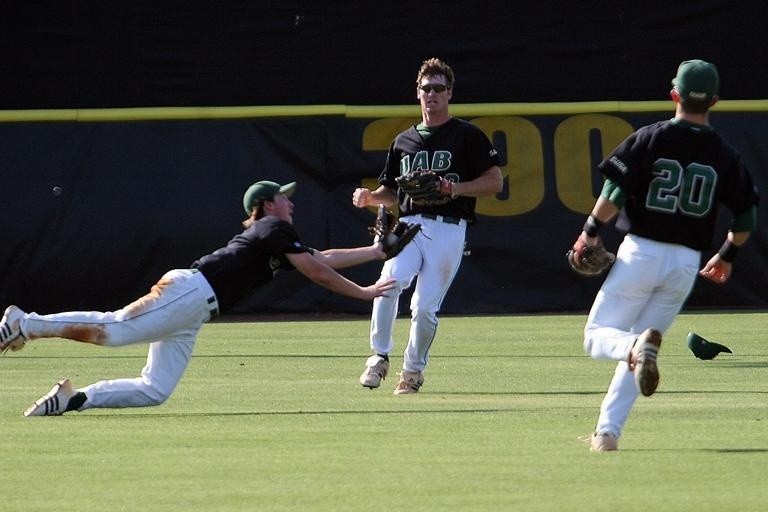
[369,204,430,260]
[566,235,615,275]
[396,169,454,205]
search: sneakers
[627,327,661,397]
[0,304,25,356]
[393,366,424,395]
[23,377,78,417]
[359,354,389,390]
[578,430,617,452]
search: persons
[0,177,399,416]
[350,57,504,396]
[565,56,757,454]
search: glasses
[418,84,451,93]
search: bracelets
[718,240,738,264]
[582,214,606,238]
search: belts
[421,212,461,225]
[191,270,218,321]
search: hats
[242,180,296,217]
[672,59,719,105]
[686,330,732,360]
